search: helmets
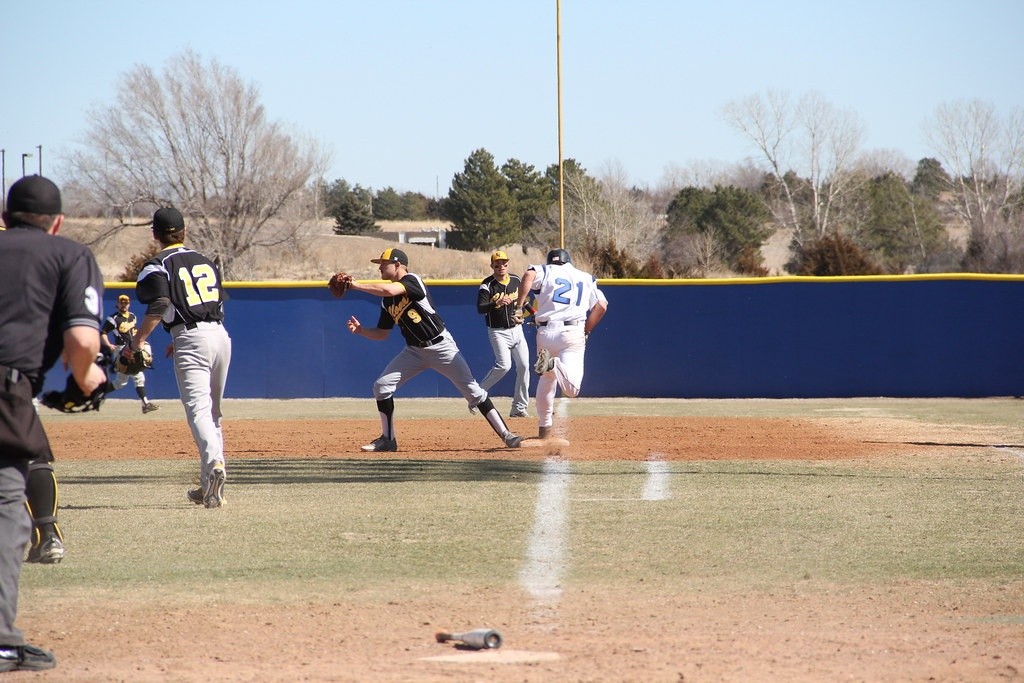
[547,248,571,264]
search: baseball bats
[435,628,504,650]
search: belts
[538,321,578,325]
[4,367,33,397]
[185,319,222,330]
[411,336,444,347]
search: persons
[100,294,163,414]
[468,250,534,418]
[25,460,64,564]
[0,173,106,671]
[510,248,609,438]
[112,205,231,507]
[328,248,522,451]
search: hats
[116,295,130,303]
[7,174,62,215]
[370,248,408,266]
[490,251,509,264]
[150,208,184,234]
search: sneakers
[142,402,160,414]
[0,642,56,673]
[539,427,553,438]
[534,348,550,376]
[510,411,531,418]
[26,537,63,565]
[202,465,227,508]
[361,433,398,452]
[502,430,523,447]
[187,487,204,504]
[469,403,479,415]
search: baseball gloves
[112,340,152,376]
[328,272,352,298]
[40,371,115,415]
